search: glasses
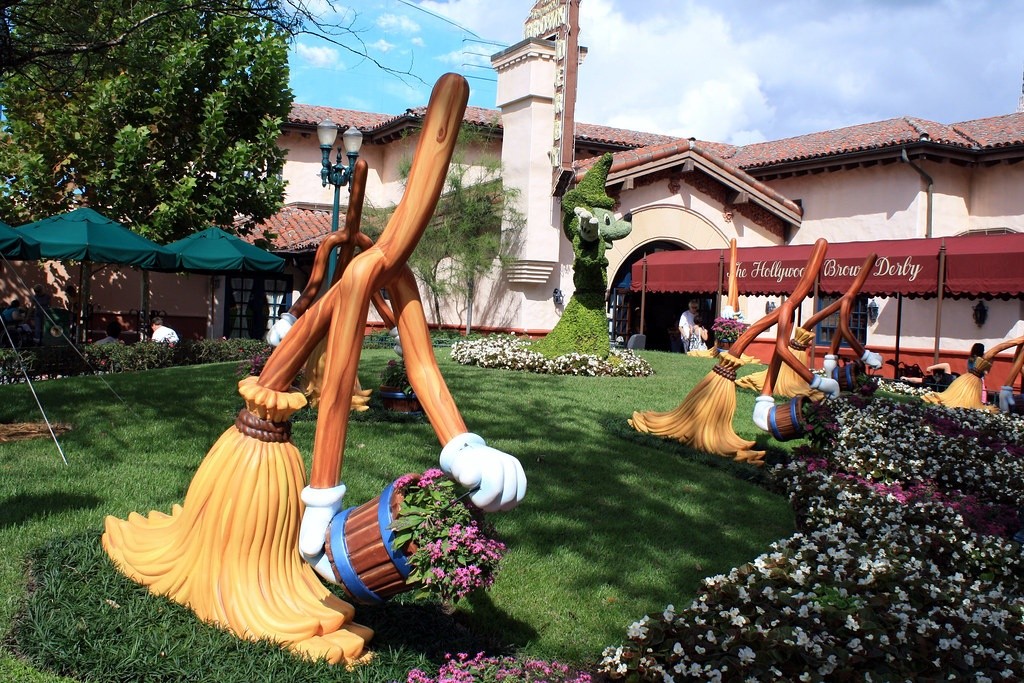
[691,306,697,309]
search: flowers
[854,372,879,398]
[800,400,840,453]
[380,359,413,396]
[711,317,747,341]
[384,468,509,604]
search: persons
[4,284,179,347]
[678,301,708,353]
[900,343,987,405]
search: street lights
[315,116,364,296]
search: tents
[0,208,285,344]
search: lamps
[553,288,563,304]
[766,301,776,314]
[971,301,988,328]
[867,300,878,325]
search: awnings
[631,233,1024,297]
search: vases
[325,473,426,605]
[832,363,860,393]
[380,385,422,417]
[768,395,812,442]
[717,338,736,352]
